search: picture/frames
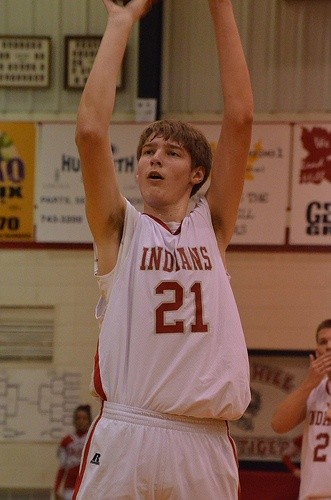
[0,35,53,89]
[64,35,128,91]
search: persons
[54,405,92,499]
[281,434,303,480]
[76,0,255,500]
[271,318,331,500]
